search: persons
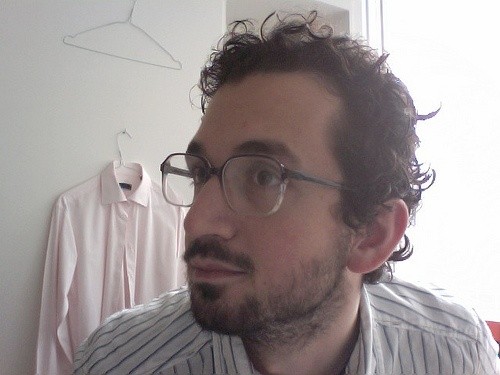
[71,9,500,375]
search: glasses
[160,153,360,217]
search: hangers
[63,0,183,71]
[112,132,142,174]
